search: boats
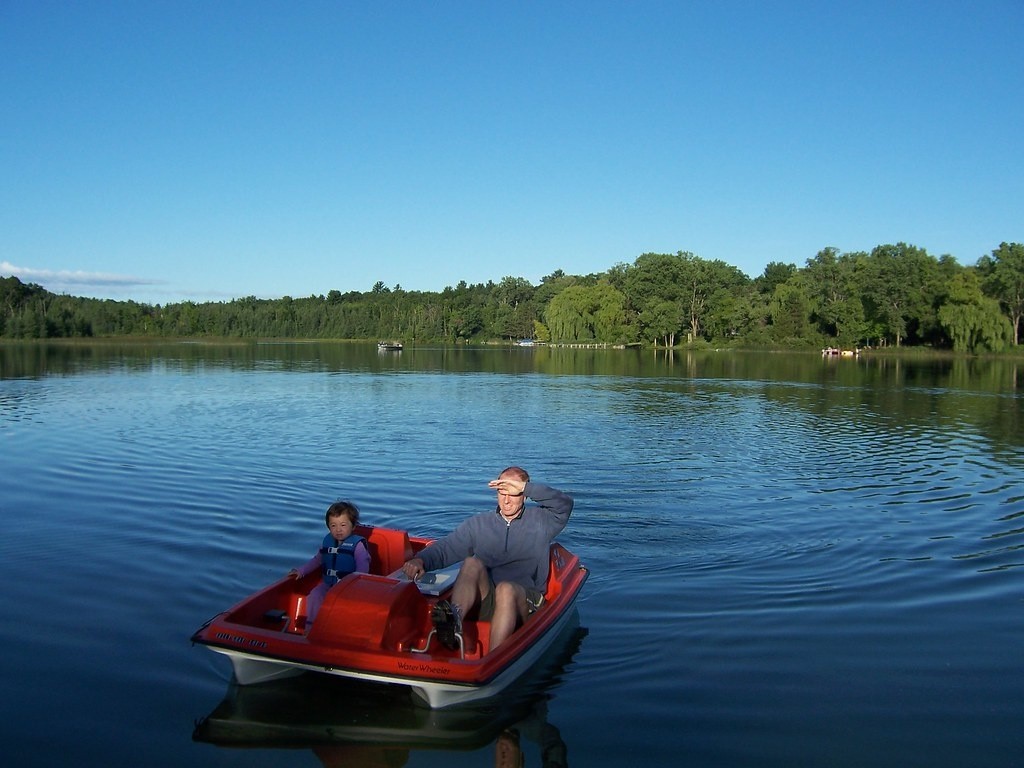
[377,341,404,351]
[191,606,580,768]
[190,522,592,709]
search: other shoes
[431,599,463,652]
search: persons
[494,704,567,768]
[401,466,575,653]
[286,501,370,637]
